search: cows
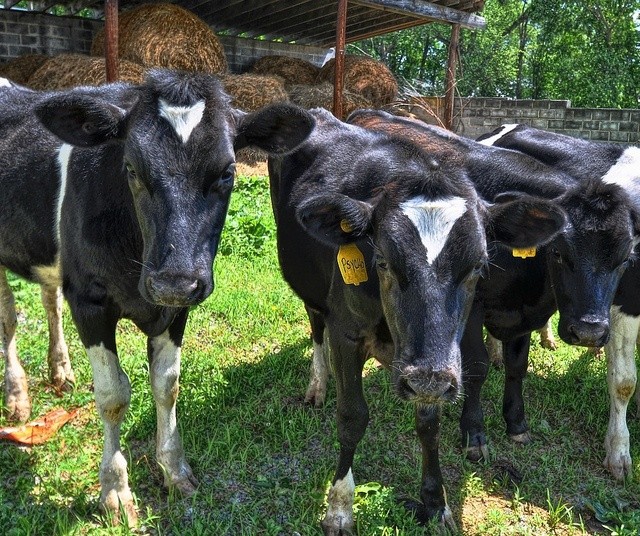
[1,70,317,533]
[345,108,639,466]
[475,123,640,484]
[267,106,567,536]
